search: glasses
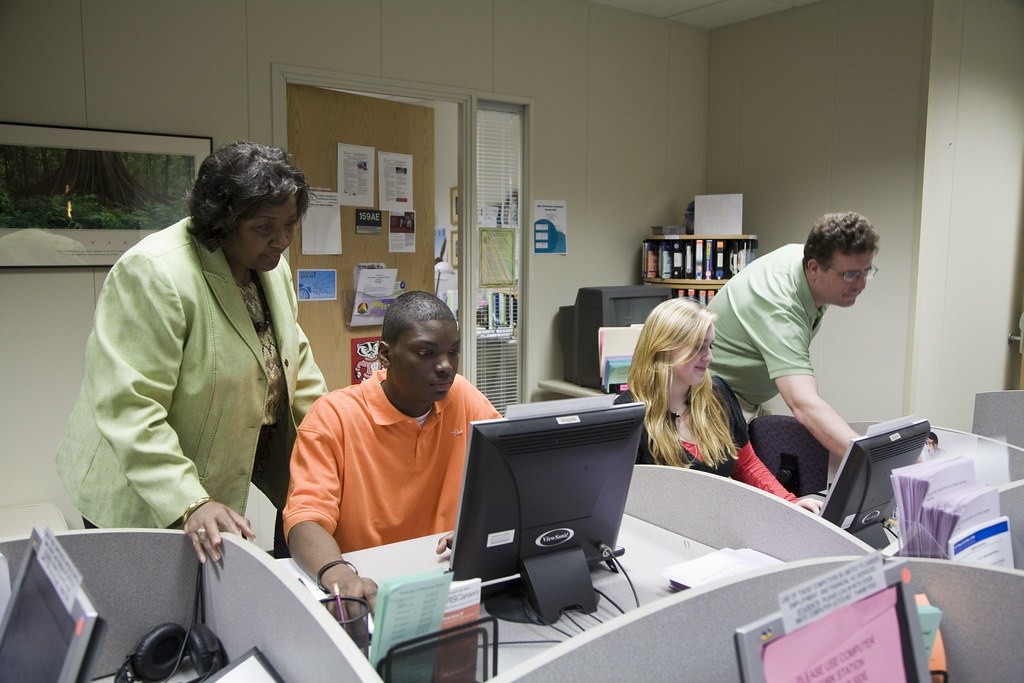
[818,259,878,282]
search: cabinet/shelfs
[642,234,760,308]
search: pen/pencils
[333,583,346,627]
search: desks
[537,379,607,400]
[0,390,1024,683]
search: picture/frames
[0,121,213,269]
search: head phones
[114,620,229,683]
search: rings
[196,528,206,535]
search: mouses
[360,599,375,641]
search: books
[671,289,719,305]
[647,239,754,281]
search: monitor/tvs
[559,284,672,391]
[0,527,108,683]
[448,401,647,626]
[819,419,931,552]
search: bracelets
[181,497,214,524]
[316,560,358,594]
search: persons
[280,291,505,617]
[924,432,947,460]
[706,212,879,458]
[613,296,824,515]
[55,141,328,562]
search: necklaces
[250,272,271,332]
